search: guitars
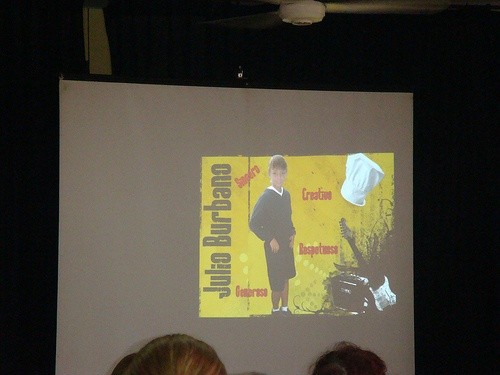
[337,218,396,311]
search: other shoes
[280,307,292,315]
[272,308,281,315]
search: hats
[340,153,384,206]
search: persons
[122,333,228,375]
[249,155,296,315]
[309,341,388,375]
